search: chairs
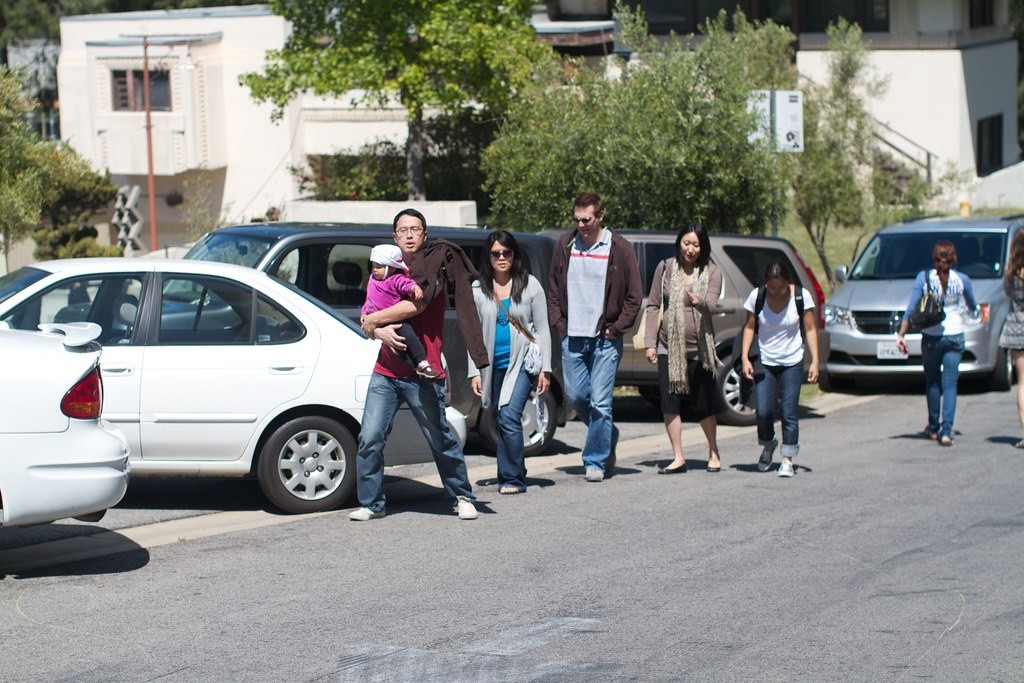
[330,261,366,306]
[106,294,138,345]
[54,303,92,334]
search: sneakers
[758,439,778,472]
[778,458,794,477]
[453,498,479,519]
[350,502,385,521]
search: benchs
[233,315,300,342]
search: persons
[359,244,440,379]
[646,223,729,475]
[997,225,1024,446]
[734,264,821,478]
[466,230,553,496]
[548,192,644,482]
[896,241,978,447]
[349,209,490,521]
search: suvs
[817,214,1024,391]
[124,221,567,459]
[535,230,831,426]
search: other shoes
[585,464,606,482]
[658,463,687,474]
[605,428,619,470]
[707,456,721,472]
[416,361,440,380]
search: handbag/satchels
[632,258,669,350]
[910,270,945,330]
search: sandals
[940,433,952,446]
[924,426,936,439]
[500,484,522,494]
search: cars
[0,321,132,528]
[0,257,467,514]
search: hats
[370,244,409,273]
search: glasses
[394,226,424,236]
[571,213,599,224]
[489,249,513,261]
